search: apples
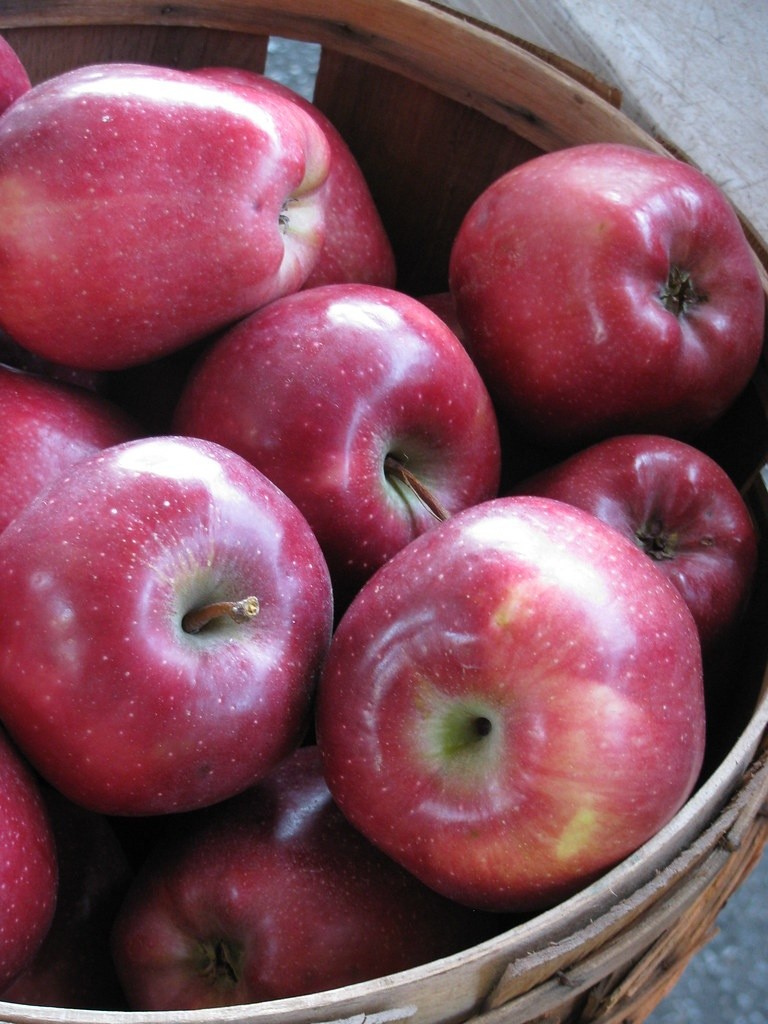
[0,29,765,1013]
